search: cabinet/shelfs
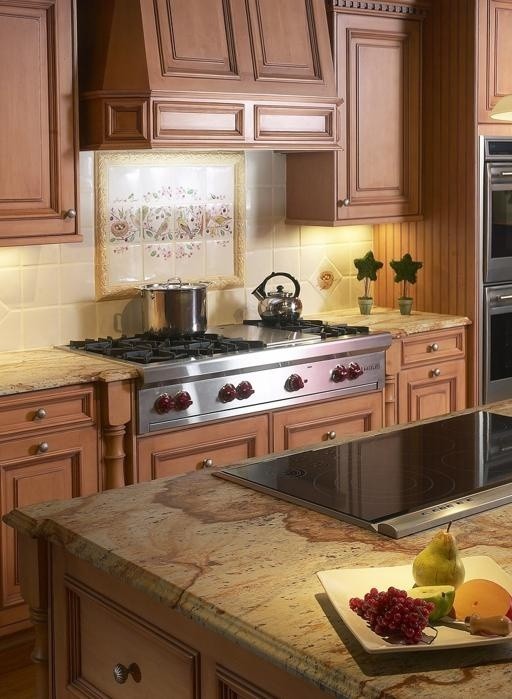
[430,0,511,126]
[286,7,432,225]
[138,413,268,482]
[212,669,274,699]
[0,3,78,241]
[143,0,249,144]
[0,387,94,440]
[395,357,463,431]
[61,579,198,699]
[271,388,385,456]
[0,424,98,637]
[248,2,335,145]
[400,327,466,368]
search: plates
[317,555,510,655]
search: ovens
[477,135,512,406]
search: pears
[412,521,464,588]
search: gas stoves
[53,319,393,435]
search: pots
[141,277,212,336]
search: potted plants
[391,252,422,313]
[354,251,383,312]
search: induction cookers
[210,409,512,541]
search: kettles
[251,270,304,324]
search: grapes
[349,586,435,645]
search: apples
[447,579,511,621]
[408,585,456,623]
